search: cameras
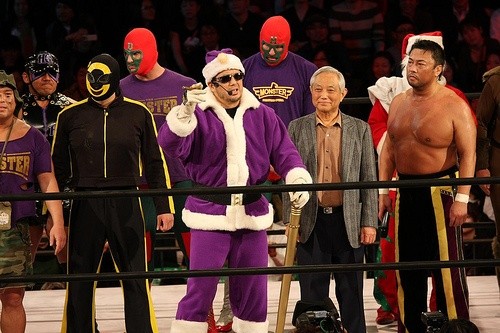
[422,310,479,333]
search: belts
[317,205,343,216]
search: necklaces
[31,94,52,101]
[1,116,15,162]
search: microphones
[213,82,232,95]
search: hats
[201,48,246,86]
[0,71,24,117]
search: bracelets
[455,192,469,203]
[379,187,389,195]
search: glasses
[210,70,245,84]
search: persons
[153,48,313,333]
[38,54,175,333]
[474,66,500,292]
[209,16,319,331]
[286,66,379,333]
[461,166,500,275]
[0,68,68,333]
[14,51,80,264]
[366,30,479,324]
[117,29,200,287]
[376,37,478,333]
[0,0,500,92]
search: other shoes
[214,318,234,332]
[375,306,399,324]
